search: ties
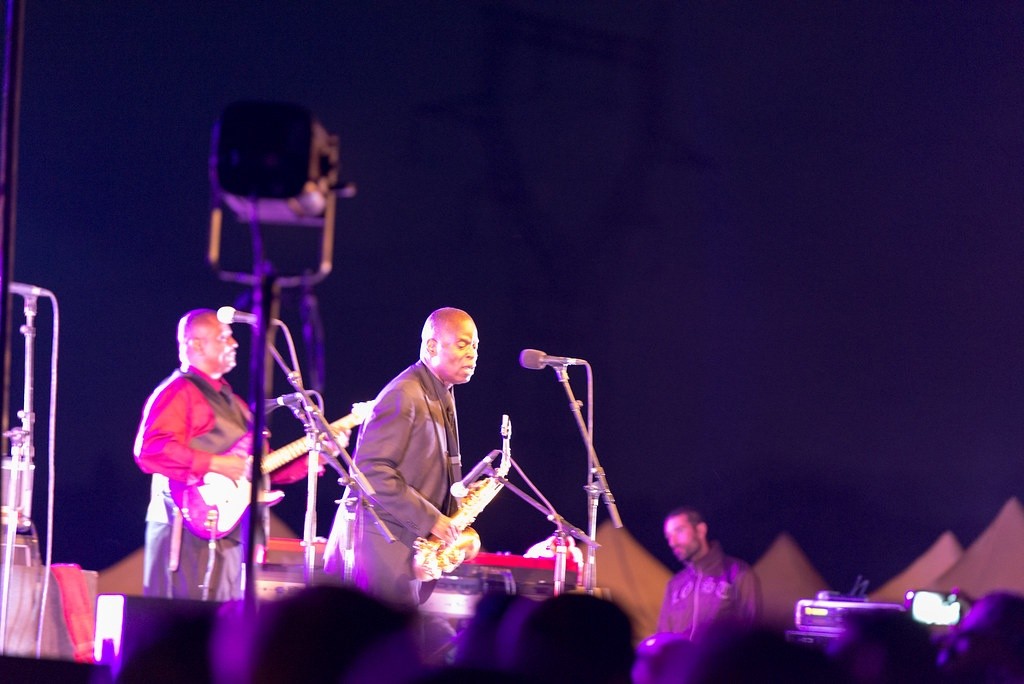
[220,389,243,421]
[447,396,456,437]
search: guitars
[170,400,378,541]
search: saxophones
[411,413,513,581]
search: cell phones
[910,591,961,626]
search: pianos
[247,547,585,597]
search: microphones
[450,450,500,497]
[12,283,49,299]
[250,390,312,416]
[217,306,282,326]
[520,349,586,369]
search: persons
[113,507,1024,684]
[322,306,480,610]
[132,308,351,605]
